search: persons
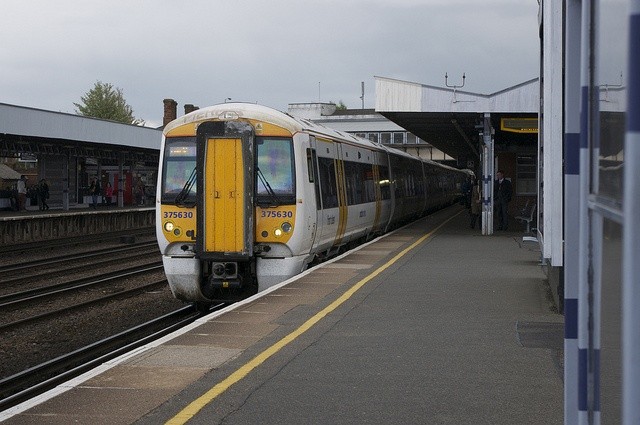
[460,178,471,209]
[494,170,513,231]
[136,177,145,206]
[39,179,49,211]
[471,178,482,230]
[104,182,112,210]
[17,175,28,212]
[90,177,100,210]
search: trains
[155,102,477,312]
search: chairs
[513,199,537,241]
